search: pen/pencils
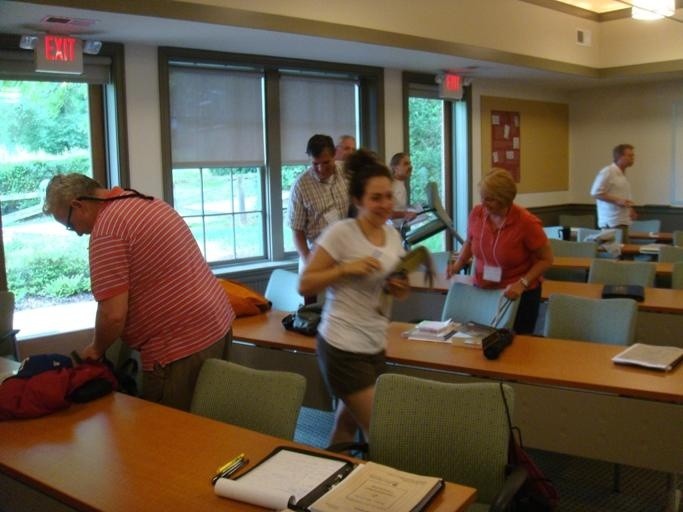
[211,452,250,485]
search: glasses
[66,206,75,231]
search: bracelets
[520,277,529,287]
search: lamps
[14,33,103,78]
[433,73,472,101]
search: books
[306,460,445,511]
[639,243,668,254]
[601,284,645,302]
[402,318,496,351]
[213,445,352,510]
[610,342,683,372]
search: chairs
[399,180,466,253]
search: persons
[387,150,418,232]
[343,148,380,217]
[287,134,352,276]
[446,166,553,335]
[590,143,640,243]
[43,172,237,410]
[296,164,411,456]
[335,135,358,161]
